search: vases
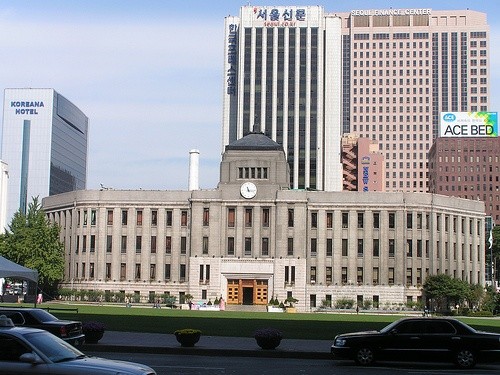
[85,328,104,344]
[254,336,283,350]
[177,333,200,347]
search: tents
[0,255,38,303]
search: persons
[111,295,115,302]
[289,303,293,308]
[424,305,430,316]
[36,290,43,305]
[97,295,103,306]
[76,296,80,304]
[126,296,132,308]
[356,306,360,314]
[186,301,193,310]
[153,296,161,309]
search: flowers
[174,328,202,337]
[256,329,282,337]
[83,320,105,333]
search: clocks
[241,182,257,198]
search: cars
[0,324,157,375]
[330,316,500,371]
[0,305,85,348]
